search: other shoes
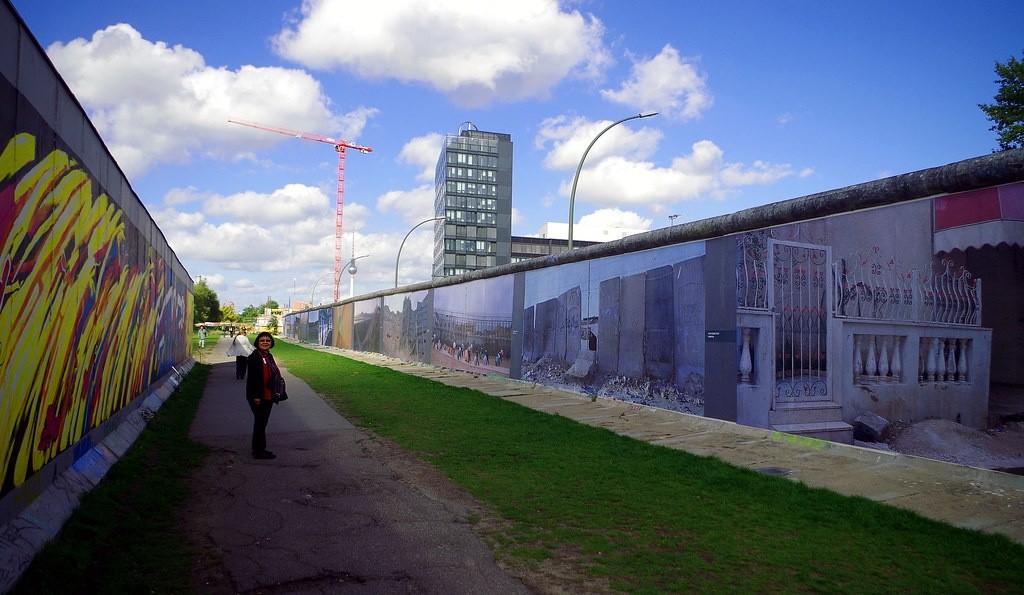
[240,376,244,379]
[251,450,276,460]
[237,376,239,379]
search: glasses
[259,338,272,342]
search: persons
[246,332,289,460]
[222,324,235,337]
[198,325,206,349]
[226,327,254,380]
[431,334,505,366]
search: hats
[200,325,204,327]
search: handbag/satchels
[268,373,285,401]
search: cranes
[227,116,374,300]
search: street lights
[334,255,369,303]
[395,216,446,288]
[567,111,659,249]
[311,272,335,307]
